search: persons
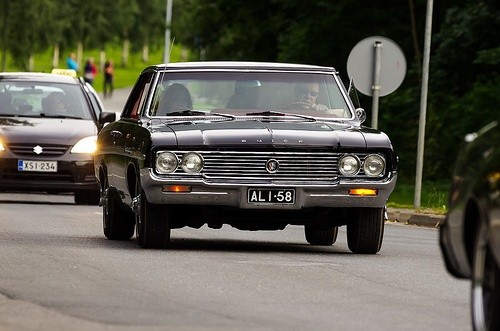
[67,53,80,72]
[47,93,70,113]
[295,79,327,111]
[104,60,114,97]
[84,58,97,84]
[162,83,193,113]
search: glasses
[300,88,318,97]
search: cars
[94,58,400,254]
[0,68,118,206]
[440,117,500,331]
